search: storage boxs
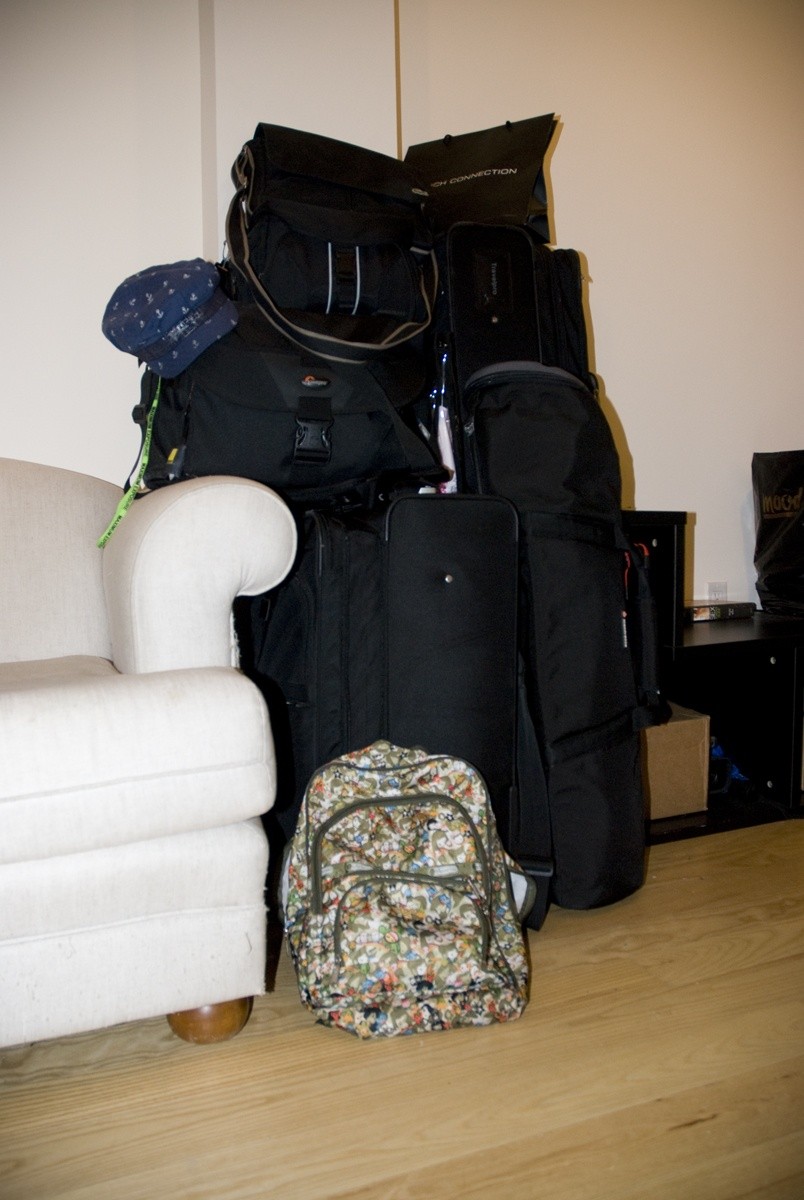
[640,700,710,819]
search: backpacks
[285,739,531,1041]
[128,121,651,924]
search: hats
[99,258,243,378]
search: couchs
[0,457,298,1049]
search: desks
[645,609,804,847]
